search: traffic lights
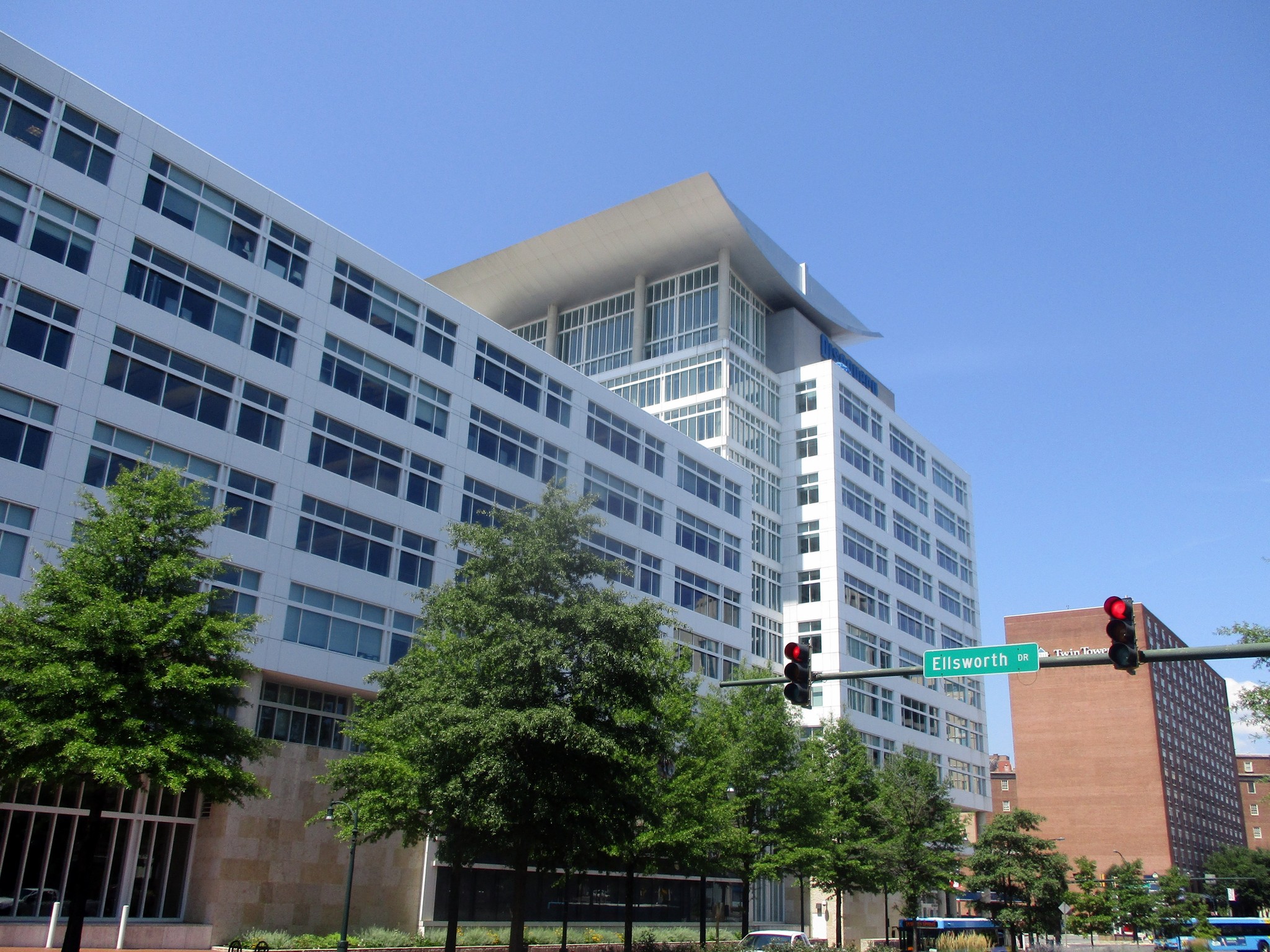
[781,642,811,706]
[1102,597,1139,672]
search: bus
[891,917,1007,952]
[1153,917,1270,952]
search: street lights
[1113,850,1139,947]
[324,797,359,952]
[1036,836,1065,950]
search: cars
[732,930,811,952]
